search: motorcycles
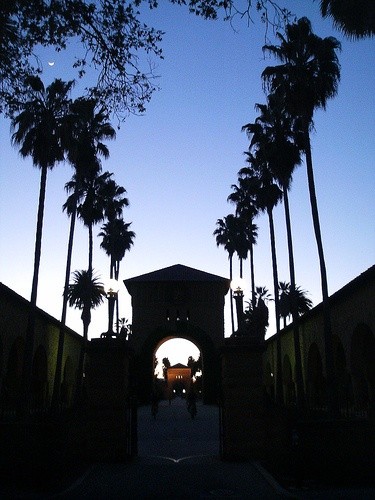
[186,397,197,420]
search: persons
[151,385,161,416]
[187,388,199,416]
